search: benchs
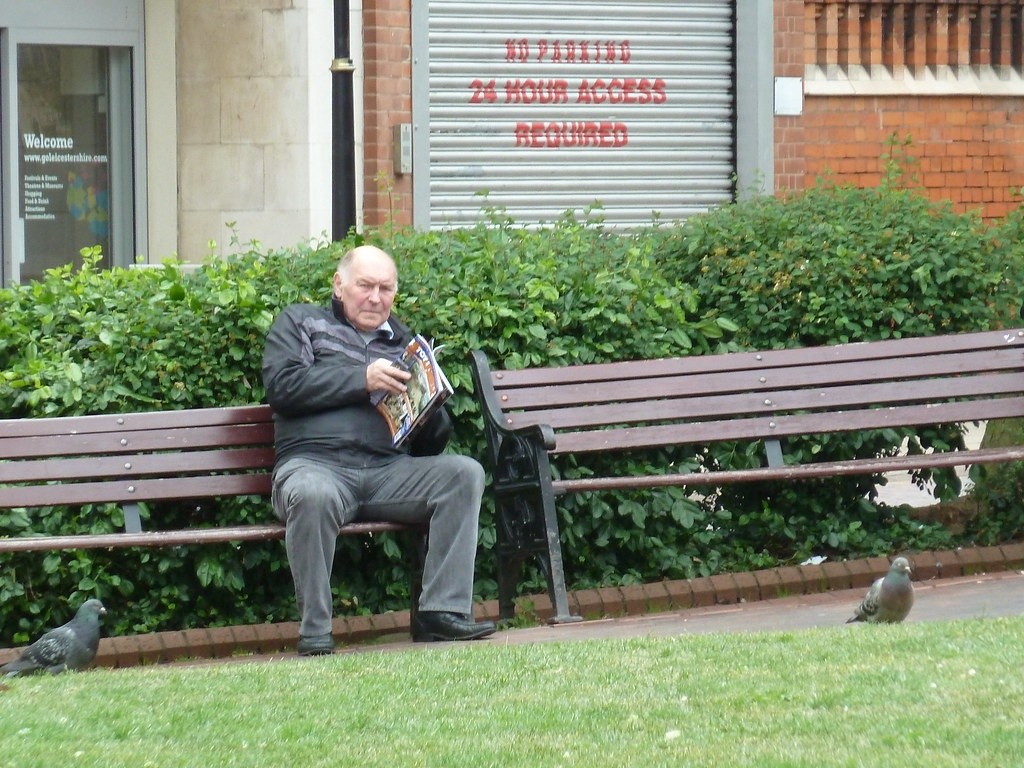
[0,403,423,635]
[465,327,1024,624]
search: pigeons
[0,597,107,676]
[846,558,914,624]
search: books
[369,333,454,449]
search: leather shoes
[411,611,497,643]
[298,635,334,654]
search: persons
[261,245,496,656]
